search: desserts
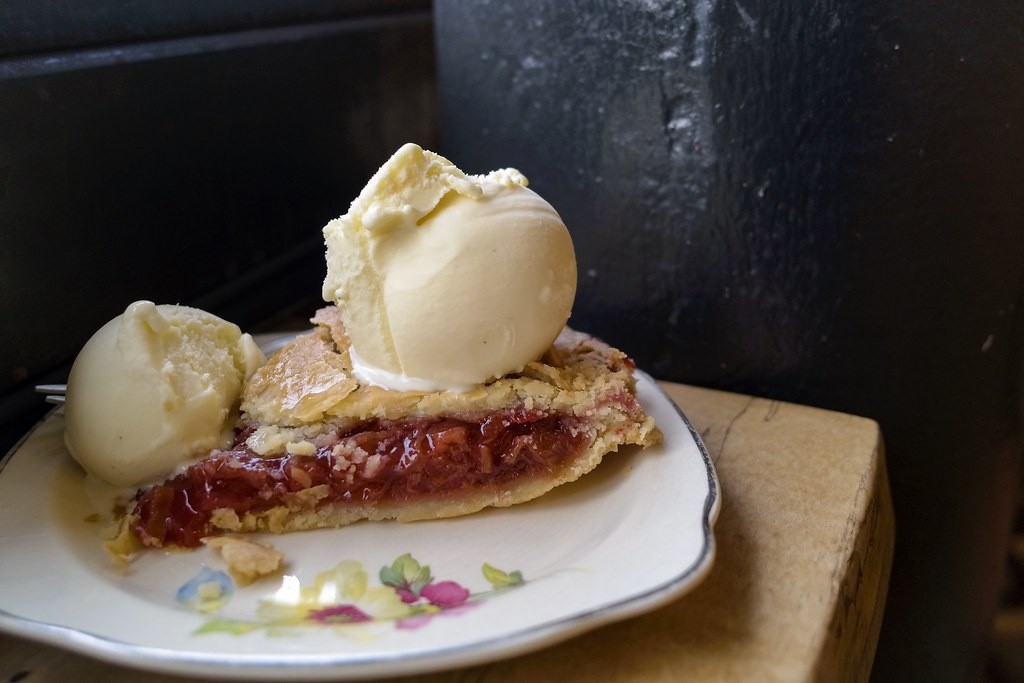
[62,300,267,486]
[321,143,577,393]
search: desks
[0,375,893,683]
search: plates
[0,325,723,681]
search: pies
[105,307,663,561]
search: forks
[35,383,67,404]
